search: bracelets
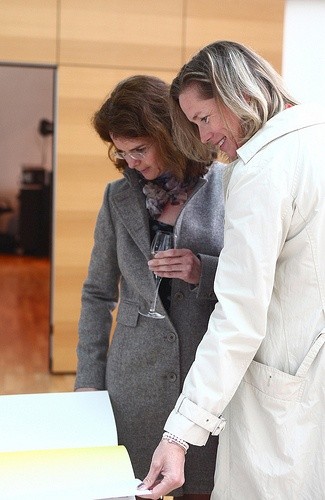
[162,431,189,454]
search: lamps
[37,119,52,184]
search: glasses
[113,140,158,161]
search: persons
[147,41,325,499]
[75,74,230,500]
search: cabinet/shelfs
[18,184,51,255]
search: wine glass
[138,231,177,318]
